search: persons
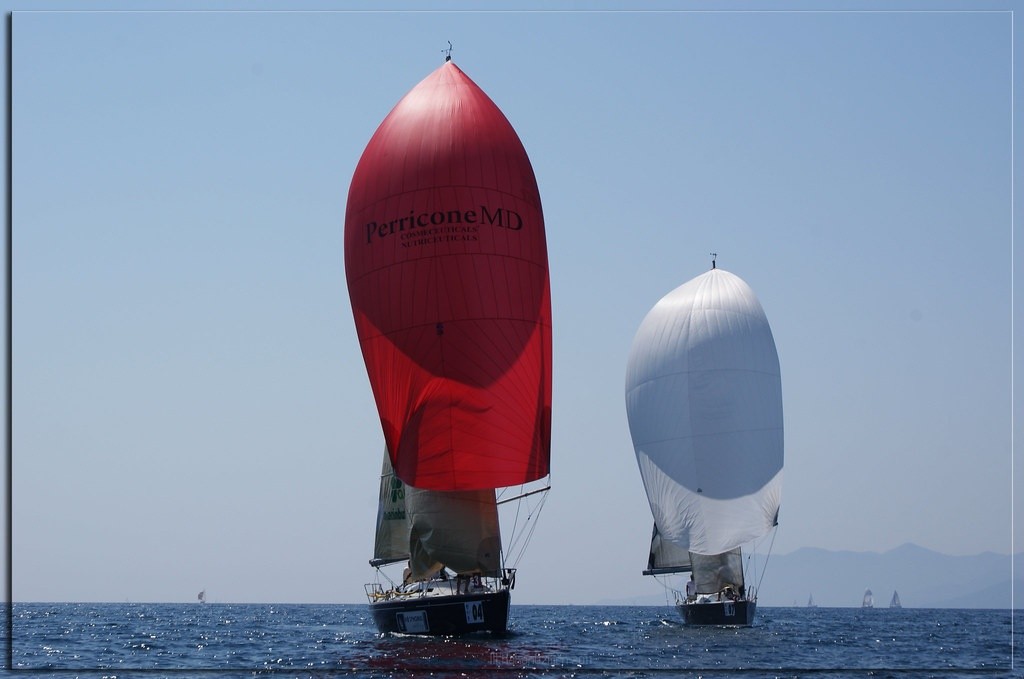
[686,574,697,603]
[403,561,414,590]
[440,566,449,579]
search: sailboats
[888,591,902,609]
[863,588,874,607]
[625,255,785,626]
[342,44,554,637]
[198,590,206,603]
